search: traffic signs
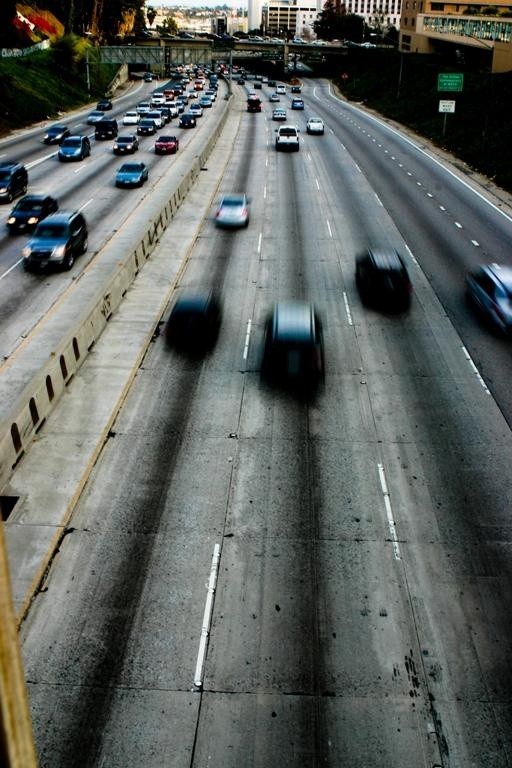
[439,100,455,113]
[438,74,463,92]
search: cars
[6,195,59,233]
[140,27,377,49]
[213,191,254,229]
[220,62,327,151]
[44,65,219,190]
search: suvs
[20,209,90,273]
[254,298,325,397]
[1,162,28,202]
[355,246,412,320]
[464,257,511,342]
[163,285,224,365]
